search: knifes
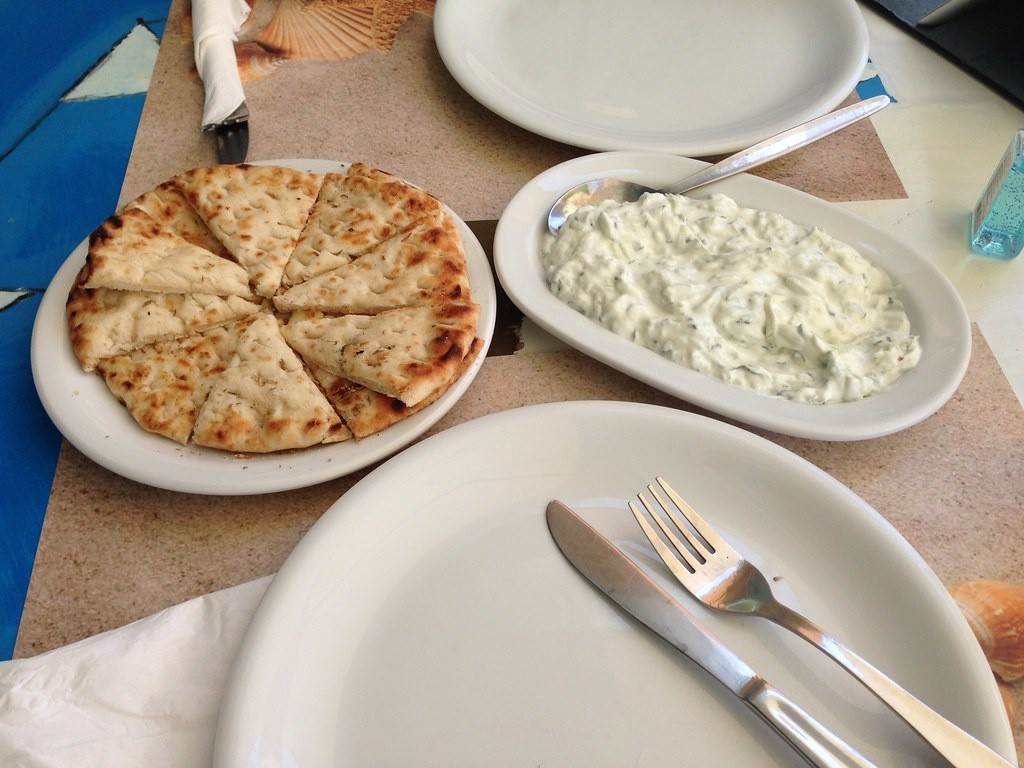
[216,122,249,167]
[545,500,881,768]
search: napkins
[0,570,280,767]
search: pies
[66,164,477,451]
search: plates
[431,0,868,158]
[29,157,499,495]
[208,400,1021,768]
[493,149,971,441]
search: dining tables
[0,0,1023,768]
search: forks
[626,475,1018,768]
[204,102,249,135]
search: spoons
[545,94,891,238]
